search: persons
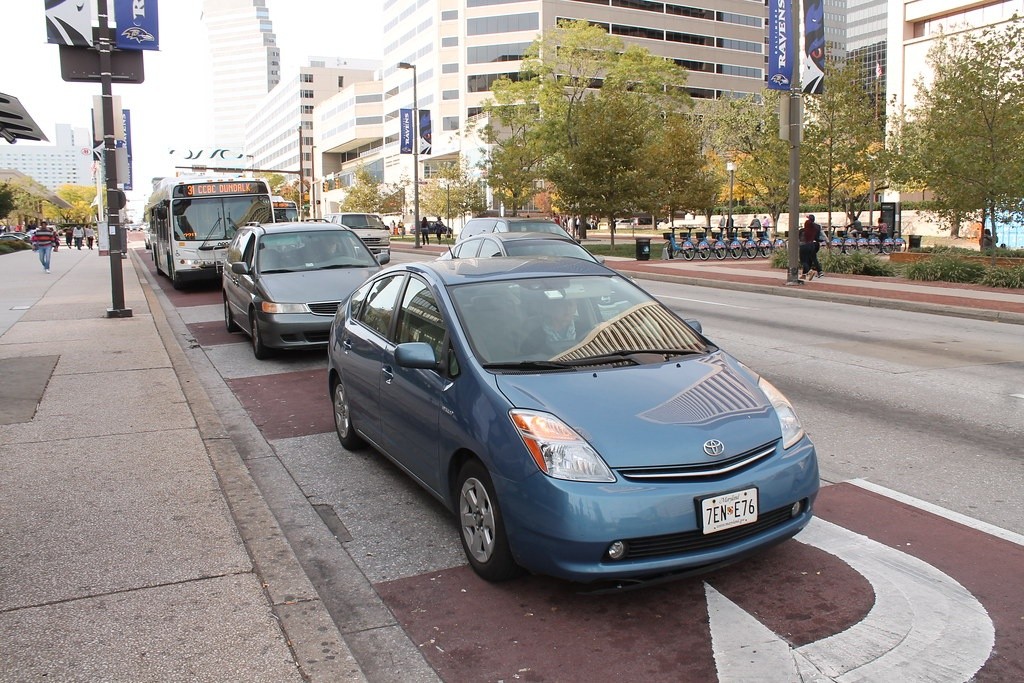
[434,216,444,244]
[979,229,997,252]
[31,223,56,274]
[798,214,830,281]
[749,214,761,235]
[546,212,599,234]
[870,218,888,244]
[389,220,406,235]
[315,236,346,263]
[1000,243,1006,255]
[762,216,769,235]
[420,216,430,245]
[847,216,863,238]
[9,223,97,253]
[613,218,616,234]
[718,213,734,235]
[520,288,610,358]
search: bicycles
[663,226,702,261]
[685,225,788,260]
[819,224,907,258]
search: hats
[806,215,815,221]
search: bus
[272,197,306,224]
[148,177,276,290]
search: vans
[325,213,392,265]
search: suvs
[218,217,387,358]
[455,216,581,254]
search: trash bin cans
[908,235,922,249]
[635,238,652,261]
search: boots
[807,269,815,281]
[800,274,806,279]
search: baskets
[663,231,869,240]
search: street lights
[397,62,423,248]
[727,161,739,238]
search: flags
[876,57,882,79]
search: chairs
[261,247,282,269]
[463,298,522,362]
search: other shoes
[45,268,50,274]
[817,271,823,278]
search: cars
[125,223,150,250]
[435,231,637,308]
[410,220,454,236]
[617,217,653,229]
[0,229,37,247]
[330,256,818,582]
